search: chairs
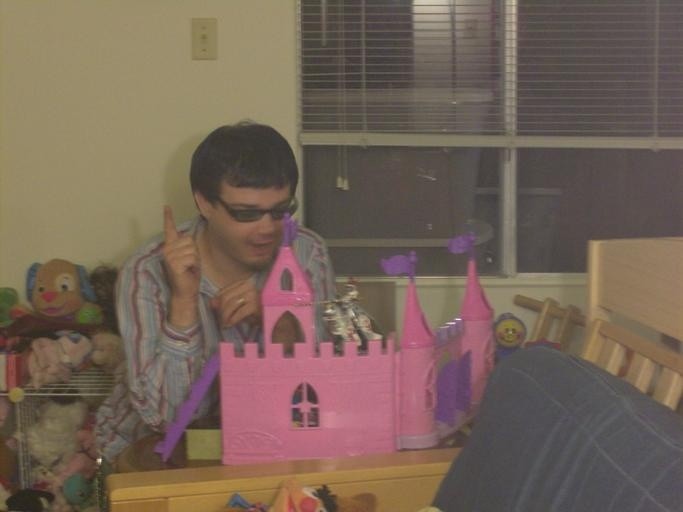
[578,318,683,412]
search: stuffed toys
[0,259,123,511]
[224,478,378,512]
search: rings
[235,294,247,307]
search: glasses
[213,196,298,222]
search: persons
[91,116,343,476]
